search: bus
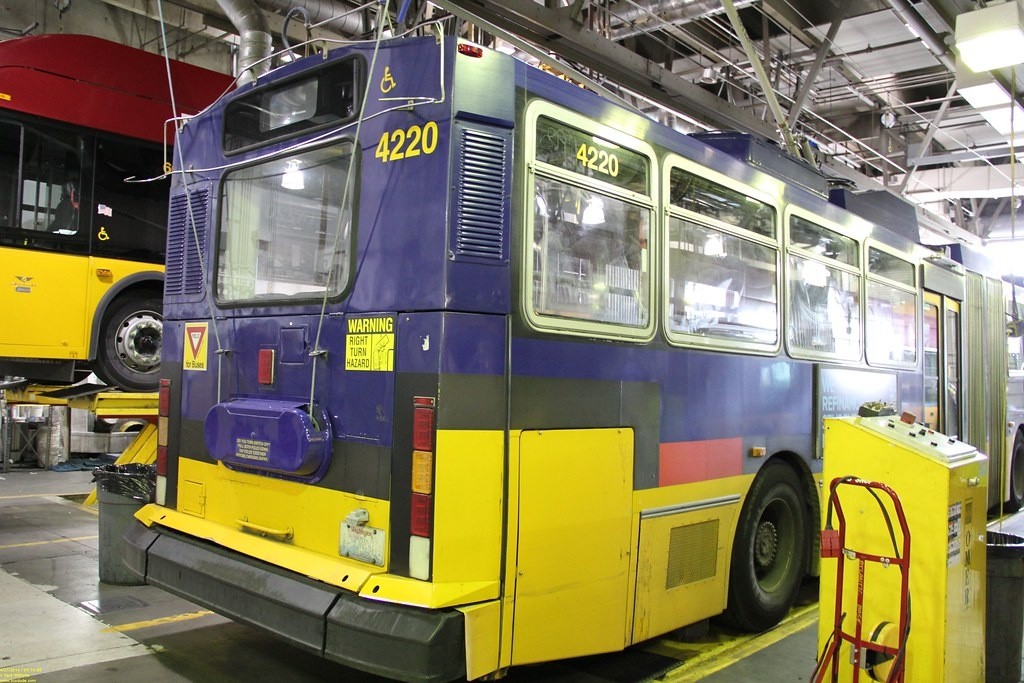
[0,32,247,393]
[116,30,1020,683]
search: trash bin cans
[93,463,157,587]
[985,529,1024,683]
